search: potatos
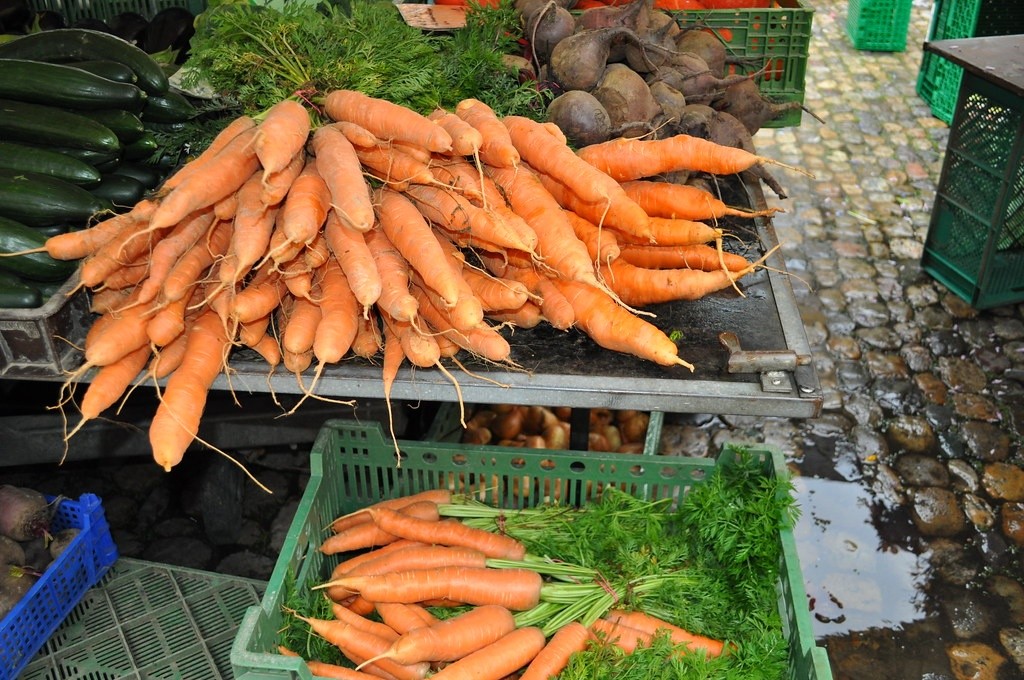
[497,0,826,200]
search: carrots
[1,82,809,680]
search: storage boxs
[915,0,980,129]
[844,0,912,53]
[228,416,834,680]
[917,1,1024,316]
[563,0,817,129]
[0,484,119,680]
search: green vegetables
[180,0,553,136]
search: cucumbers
[0,28,201,308]
